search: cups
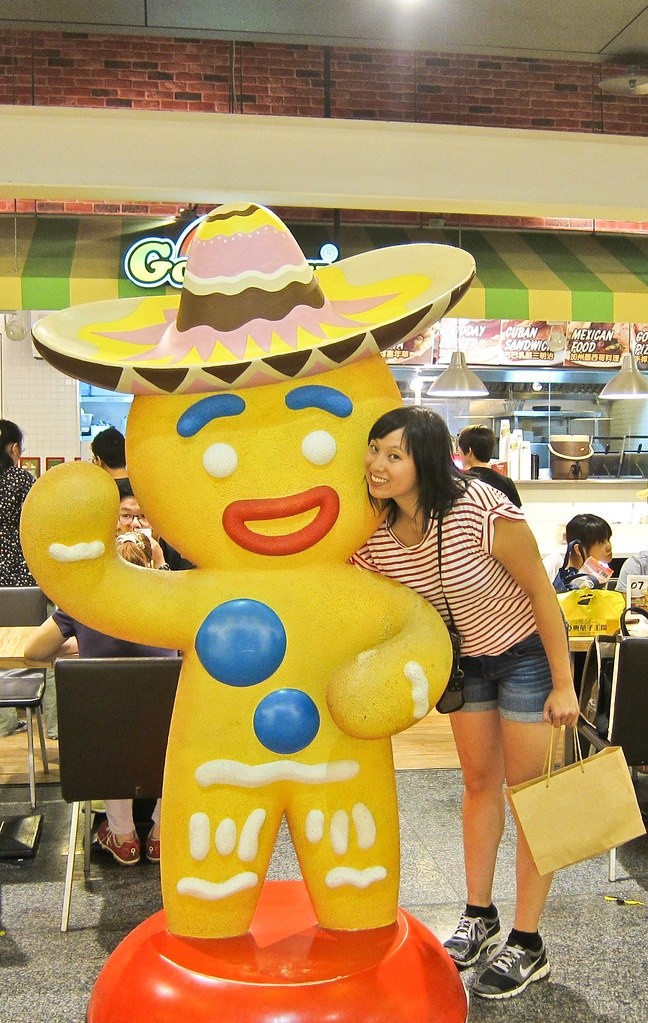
[138,528,151,539]
[498,419,531,480]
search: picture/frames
[20,457,40,479]
[46,457,65,471]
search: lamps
[426,318,489,397]
[597,323,648,399]
[5,314,30,341]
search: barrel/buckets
[546,434,595,480]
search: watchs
[156,564,170,572]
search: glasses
[92,458,98,465]
[117,514,151,528]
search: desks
[0,626,52,860]
[566,636,593,652]
[55,634,155,852]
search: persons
[0,420,58,739]
[92,426,135,502]
[458,424,522,510]
[347,406,580,1000]
[81,479,197,814]
[614,549,648,608]
[542,514,612,591]
[24,532,183,865]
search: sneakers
[472,935,551,999]
[443,911,501,966]
[96,820,140,864]
[0,718,27,736]
[145,823,160,861]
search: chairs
[565,558,647,882]
[1,586,184,873]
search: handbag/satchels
[434,670,465,715]
[504,724,646,876]
[552,539,599,593]
[558,588,626,636]
